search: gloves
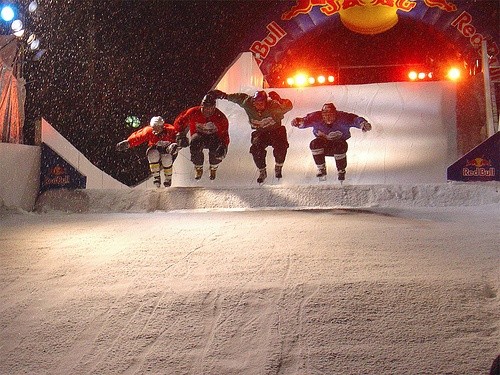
[115,140,130,152]
[214,147,226,160]
[176,134,189,147]
[166,144,181,155]
[360,120,372,133]
[268,91,283,106]
[291,118,304,128]
[206,90,226,100]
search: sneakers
[257,168,267,187]
[194,165,204,179]
[210,168,217,180]
[163,179,171,187]
[152,176,161,188]
[275,165,283,181]
[338,173,345,185]
[315,168,327,181]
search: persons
[116,116,181,188]
[291,103,372,185]
[208,89,293,188]
[173,94,231,184]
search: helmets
[253,90,268,111]
[150,116,165,135]
[201,94,216,117]
[322,103,337,124]
[123,116,141,130]
